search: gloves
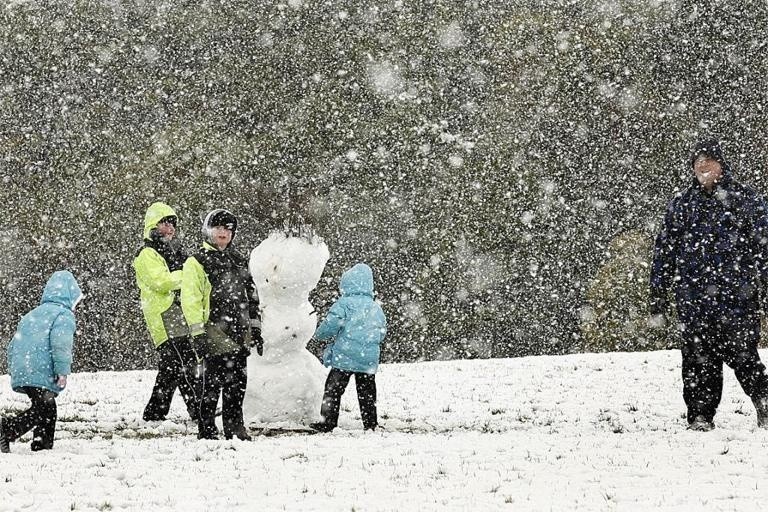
[248,327,264,355]
[192,333,212,359]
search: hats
[211,211,236,231]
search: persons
[181,209,263,440]
[134,202,225,422]
[0,270,84,453]
[647,140,768,432]
[308,263,388,433]
